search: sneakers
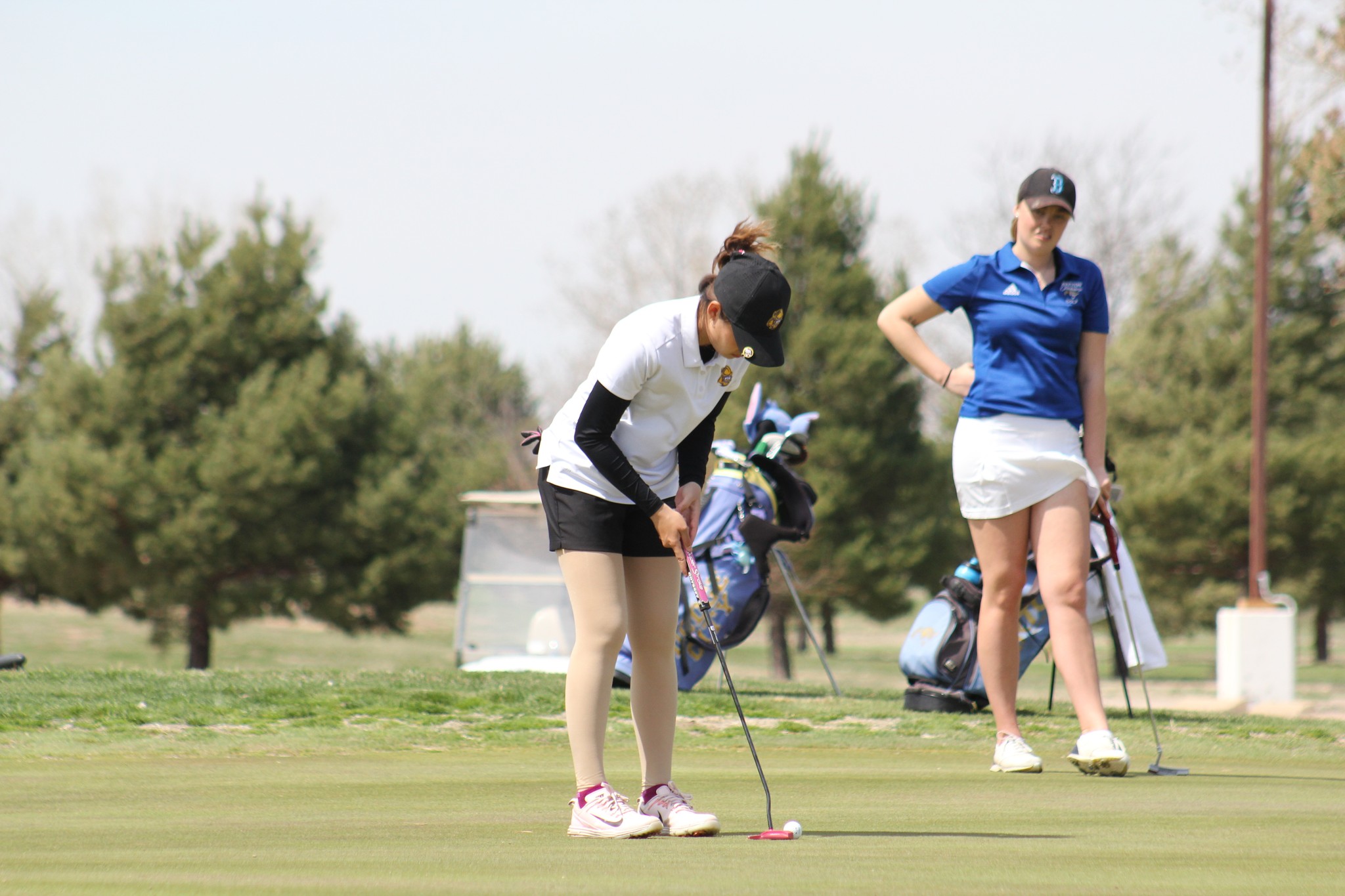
[567,782,664,840]
[636,781,720,836]
[1066,729,1130,777]
[989,731,1042,773]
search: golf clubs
[771,543,842,697]
[1096,492,1190,775]
[683,544,794,841]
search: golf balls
[783,821,802,840]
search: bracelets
[943,367,953,387]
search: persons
[876,169,1135,775]
[536,220,791,838]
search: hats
[713,252,791,367]
[1017,168,1076,222]
